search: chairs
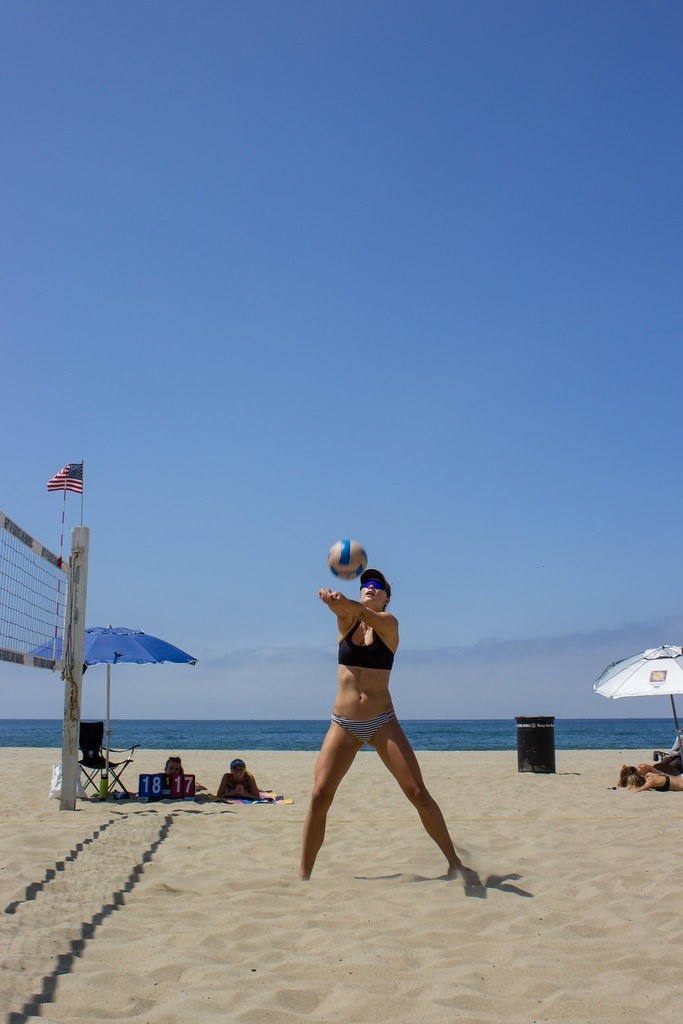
[78,721,140,800]
[653,728,683,762]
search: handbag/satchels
[49,763,86,799]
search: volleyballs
[327,539,369,580]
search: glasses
[360,580,387,591]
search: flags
[47,463,82,494]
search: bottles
[99,773,109,800]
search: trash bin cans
[513,716,557,773]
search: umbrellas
[25,624,200,774]
[592,645,683,760]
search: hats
[361,569,390,597]
[231,760,245,769]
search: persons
[217,759,261,800]
[299,569,461,880]
[618,763,683,793]
[158,756,208,792]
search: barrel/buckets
[514,717,556,774]
